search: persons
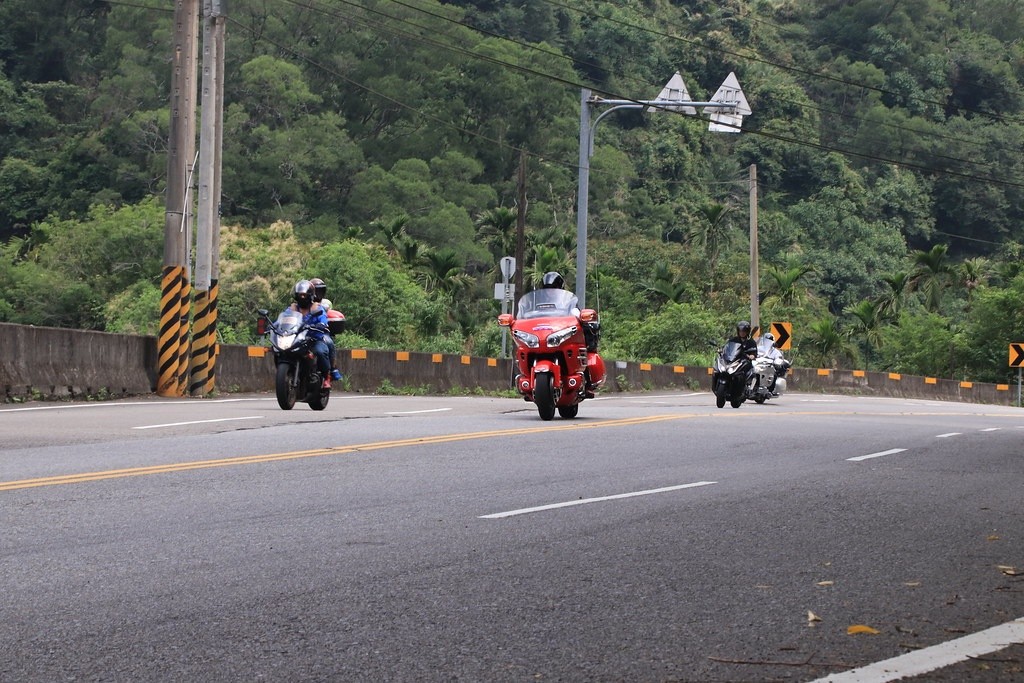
[716,321,758,359]
[523,272,594,399]
[264,277,341,388]
[757,333,781,359]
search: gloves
[265,327,270,334]
[316,323,327,330]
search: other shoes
[585,389,594,397]
[524,396,532,401]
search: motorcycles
[708,340,759,409]
[255,304,346,411]
[746,337,788,405]
[498,253,607,421]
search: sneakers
[331,371,342,381]
[323,375,332,388]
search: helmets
[737,322,751,338]
[295,280,315,308]
[310,278,327,302]
[541,272,565,300]
[763,333,774,347]
